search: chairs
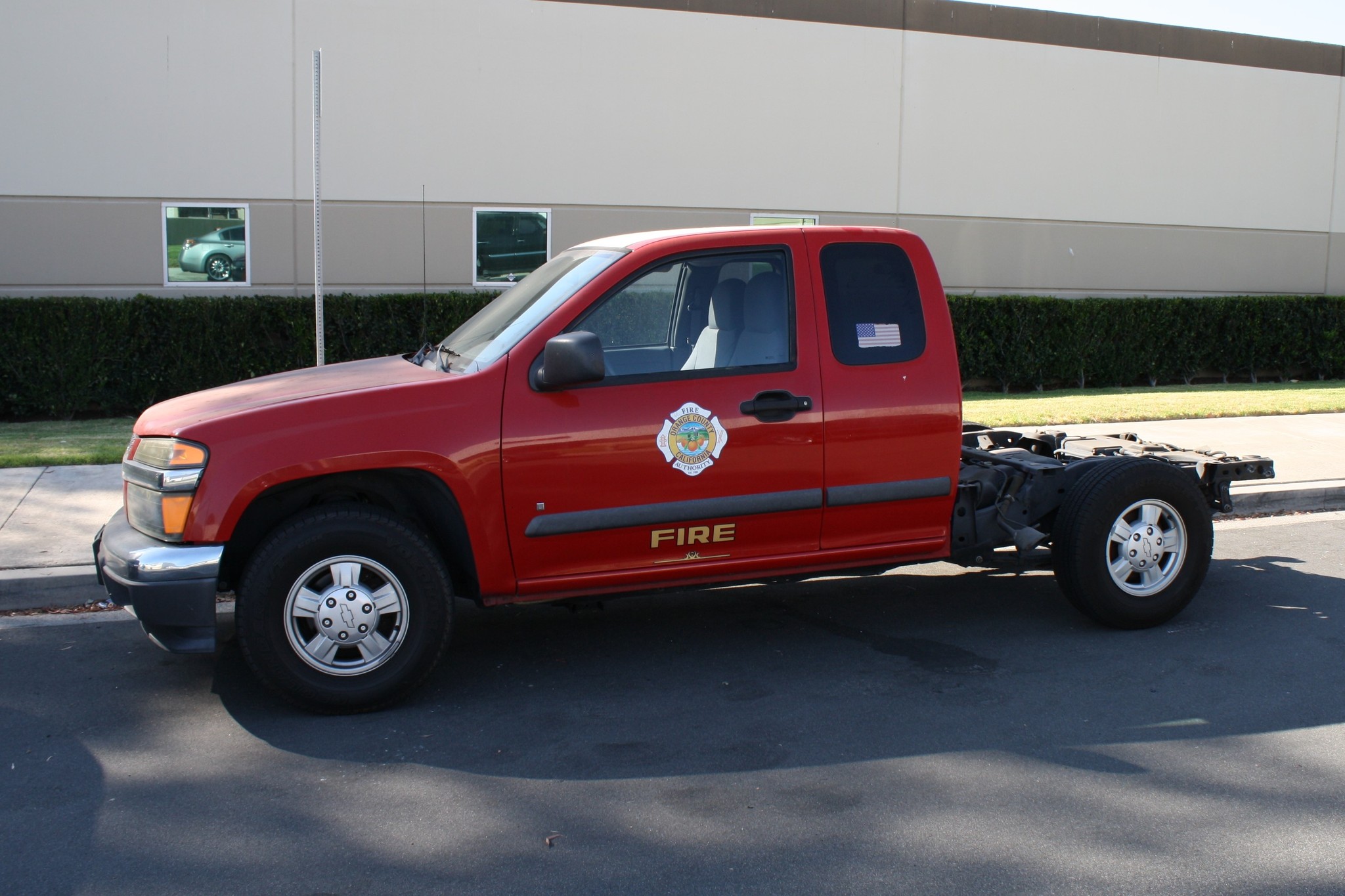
[681,278,745,370]
[729,271,788,365]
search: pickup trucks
[90,224,1280,720]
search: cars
[177,224,245,282]
[477,211,547,277]
